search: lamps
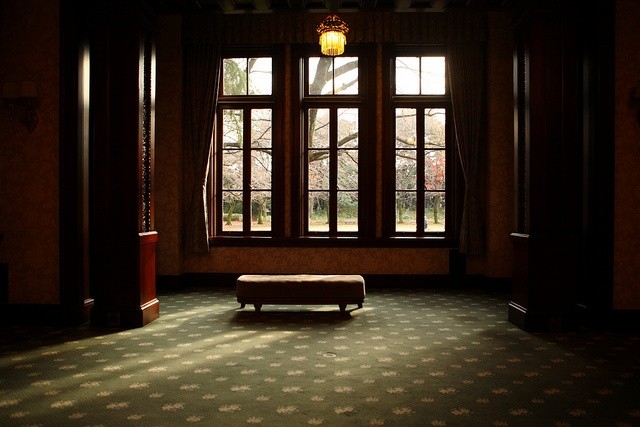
[1,80,41,134]
[315,10,350,55]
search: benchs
[236,274,365,315]
